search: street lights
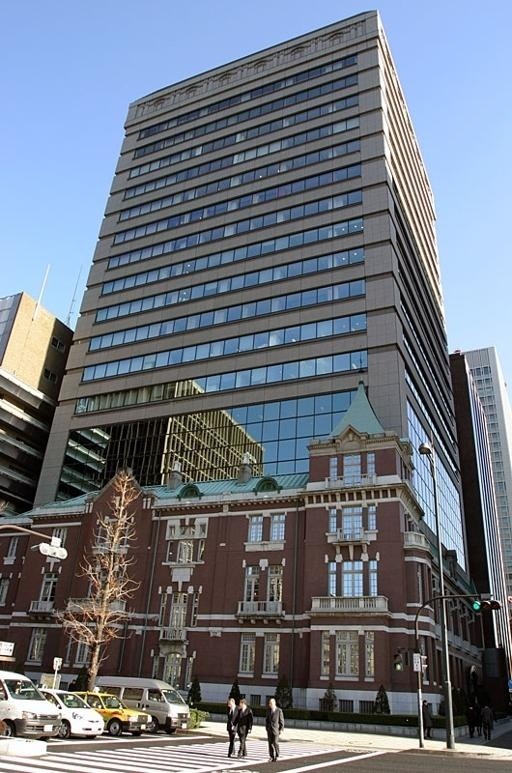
[416,441,455,749]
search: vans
[1,670,63,741]
[94,675,192,735]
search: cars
[77,691,154,735]
[21,688,105,739]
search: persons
[466,702,496,741]
[265,699,285,763]
[232,698,252,758]
[423,699,434,738]
[225,698,238,758]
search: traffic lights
[472,598,502,612]
[393,646,405,672]
